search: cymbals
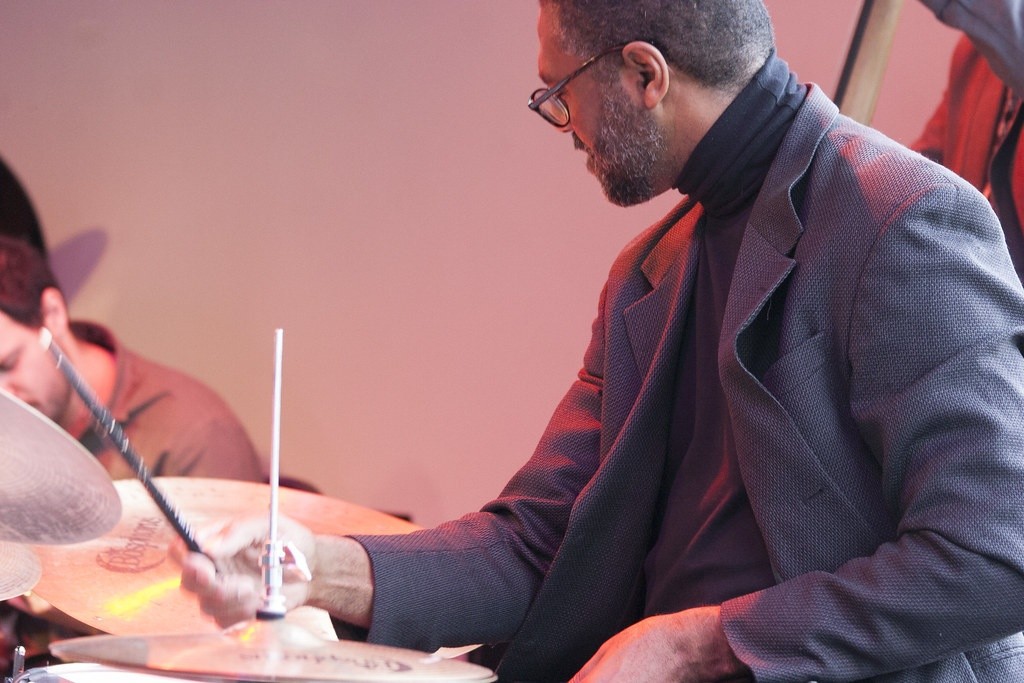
[26,475,431,641]
[48,620,499,683]
[1,387,122,547]
[0,542,43,601]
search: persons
[170,0,1024,683]
[0,227,266,670]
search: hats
[0,153,56,328]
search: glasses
[527,40,654,128]
